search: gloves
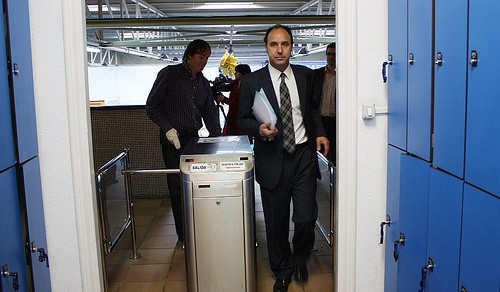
[165,128,181,149]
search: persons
[218,64,251,136]
[239,25,329,291]
[311,42,336,163]
[147,38,222,247]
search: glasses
[326,53,335,56]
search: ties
[280,73,295,155]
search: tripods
[213,101,227,122]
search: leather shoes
[273,274,291,292]
[292,253,308,283]
